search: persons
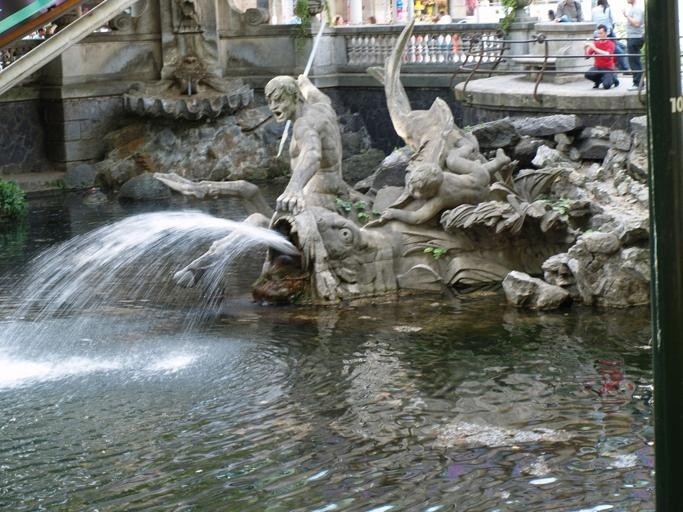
[383,145,512,225]
[623,0,644,90]
[33,27,46,40]
[585,24,619,88]
[555,0,582,22]
[264,73,342,217]
[330,15,343,26]
[592,0,615,37]
[430,9,452,24]
[367,16,376,23]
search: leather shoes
[615,80,619,87]
[593,81,601,88]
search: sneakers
[628,84,639,90]
